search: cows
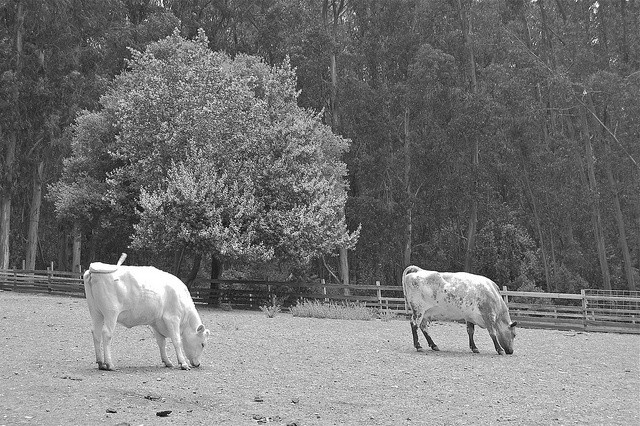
[401,264,517,356]
[83,252,210,371]
